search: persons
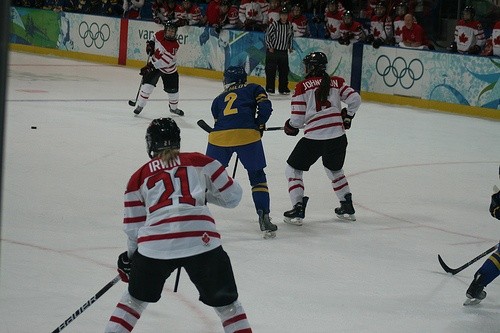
[449,4,500,56]
[134,20,184,116]
[466,191,500,302]
[265,7,294,93]
[206,65,277,231]
[106,117,253,333]
[123,0,427,49]
[283,51,362,219]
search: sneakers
[463,273,487,306]
[283,196,309,226]
[257,209,278,240]
[168,104,184,117]
[334,193,356,221]
[133,105,144,117]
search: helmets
[164,20,178,31]
[145,117,181,158]
[303,52,328,71]
[222,65,247,90]
[279,7,288,14]
[293,0,475,27]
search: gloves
[139,62,154,75]
[341,107,356,130]
[146,40,155,56]
[336,32,481,58]
[215,25,223,34]
[117,251,132,282]
[284,118,299,136]
[255,118,267,137]
[176,19,189,27]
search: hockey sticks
[52,274,121,333]
[128,51,151,106]
[232,154,239,178]
[174,268,182,292]
[197,119,284,133]
[438,241,499,275]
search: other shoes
[266,89,275,96]
[283,90,290,95]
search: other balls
[31,125,36,130]
